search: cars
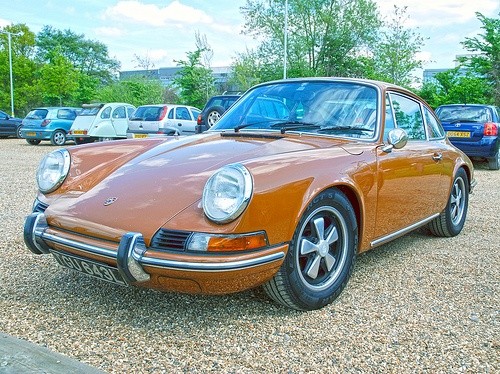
[22,108,80,145]
[66,102,135,144]
[23,77,476,310]
[128,104,202,139]
[0,108,25,139]
[435,103,500,170]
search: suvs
[195,90,296,134]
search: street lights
[0,28,24,117]
